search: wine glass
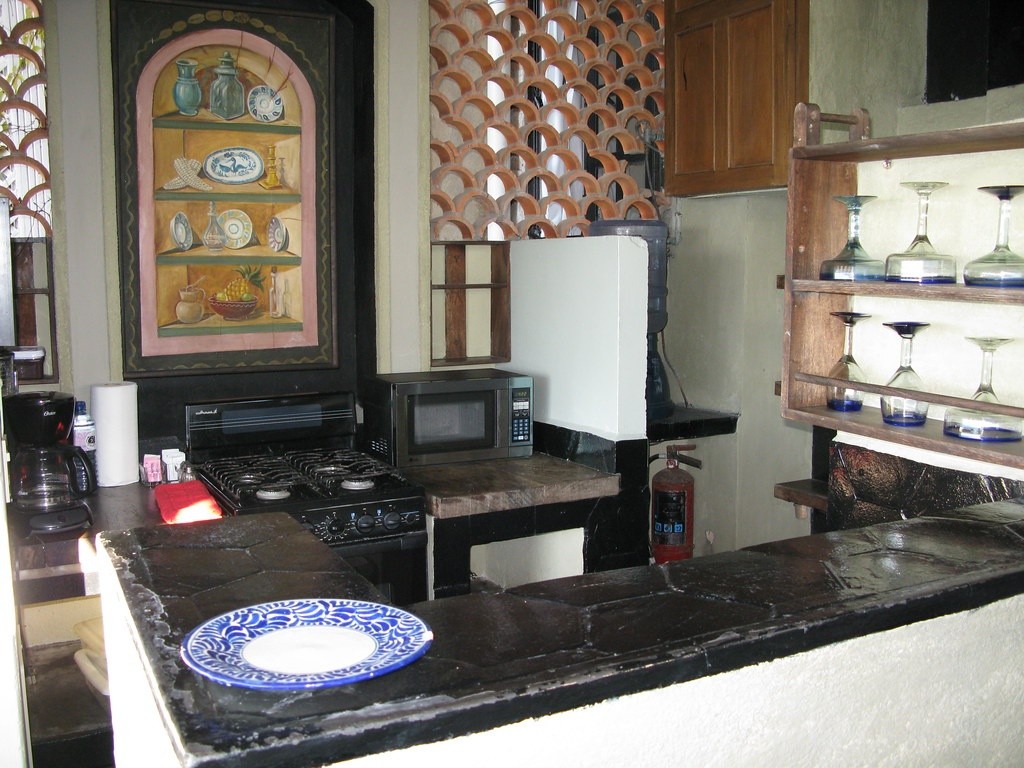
[880,323,933,426]
[818,196,885,280]
[964,185,1023,289]
[886,181,957,283]
[943,338,1023,441]
[827,311,873,412]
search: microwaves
[364,369,534,469]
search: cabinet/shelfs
[781,102,1024,481]
[662,0,810,198]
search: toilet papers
[89,381,140,488]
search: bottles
[588,220,669,332]
[73,401,98,493]
[0,349,14,397]
[175,461,196,483]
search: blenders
[2,391,97,538]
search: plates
[179,598,434,688]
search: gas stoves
[181,390,427,544]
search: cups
[139,461,165,486]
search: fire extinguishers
[647,441,704,565]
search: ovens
[332,532,429,605]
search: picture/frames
[111,1,339,379]
